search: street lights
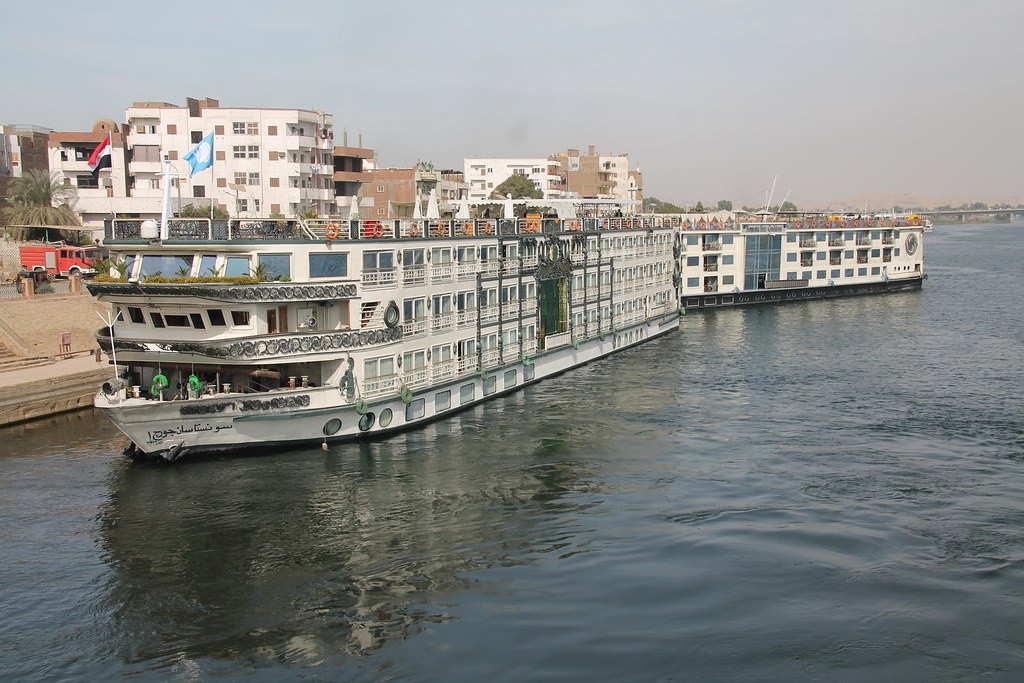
[218,189,241,219]
[109,176,125,218]
[166,160,181,218]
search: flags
[87,134,112,181]
[182,131,213,179]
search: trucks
[19,239,103,279]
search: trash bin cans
[17,270,48,295]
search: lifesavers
[573,339,580,350]
[825,221,830,228]
[355,401,369,415]
[599,333,605,341]
[682,222,688,230]
[612,328,618,337]
[481,369,490,381]
[700,222,705,230]
[326,224,338,239]
[569,222,580,231]
[522,355,530,366]
[438,224,446,236]
[867,220,873,226]
[647,318,650,326]
[854,219,859,227]
[485,223,492,234]
[401,388,413,404]
[681,307,686,315]
[462,223,470,235]
[373,223,384,238]
[811,221,816,228]
[840,221,845,227]
[626,221,631,228]
[880,219,886,226]
[795,221,801,229]
[893,219,899,225]
[409,222,419,237]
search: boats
[680,213,933,310]
[921,219,934,232]
[85,188,681,464]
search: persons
[483,208,490,219]
[615,209,622,217]
